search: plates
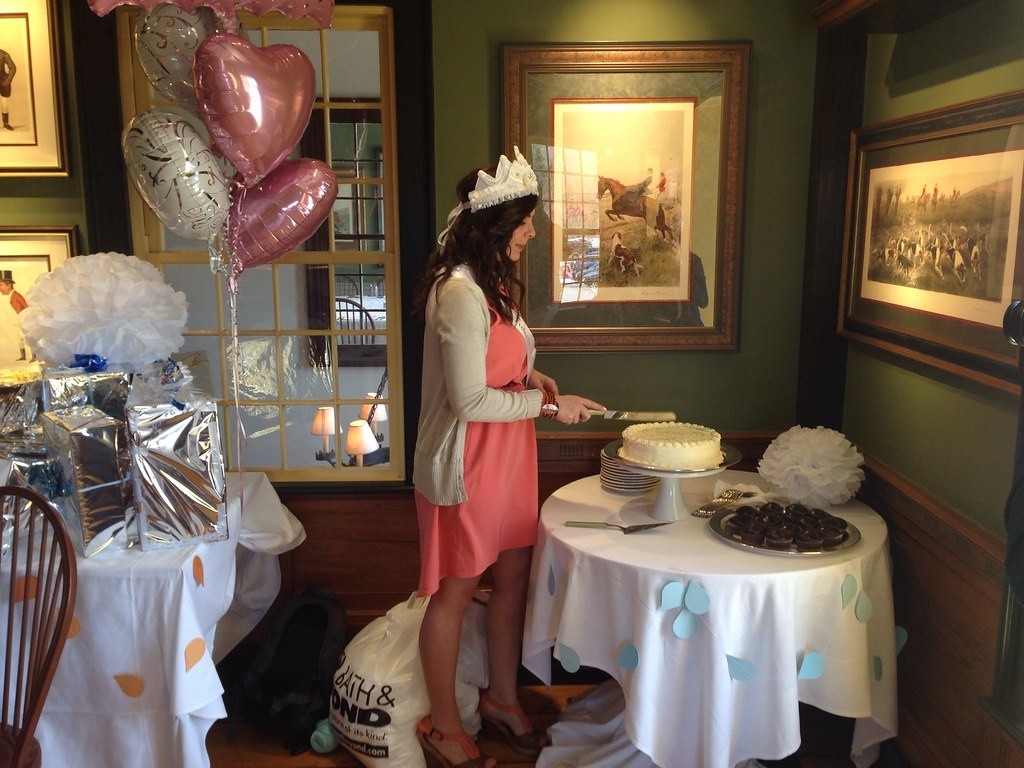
[600,448,662,493]
[707,501,862,558]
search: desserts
[729,501,847,551]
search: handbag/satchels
[328,586,490,768]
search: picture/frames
[835,87,1024,397]
[0,0,73,181]
[498,34,754,352]
[0,224,79,378]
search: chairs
[336,275,361,295]
[336,299,376,344]
[0,485,77,768]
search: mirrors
[300,98,387,369]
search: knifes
[588,409,677,422]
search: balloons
[122,110,229,240]
[193,33,317,191]
[133,3,253,108]
[222,156,338,271]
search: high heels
[475,693,548,756]
[416,713,495,768]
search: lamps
[310,392,389,467]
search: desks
[0,470,268,768]
[333,294,386,343]
[524,474,900,768]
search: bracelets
[542,393,560,420]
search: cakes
[617,420,724,470]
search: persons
[411,144,606,768]
